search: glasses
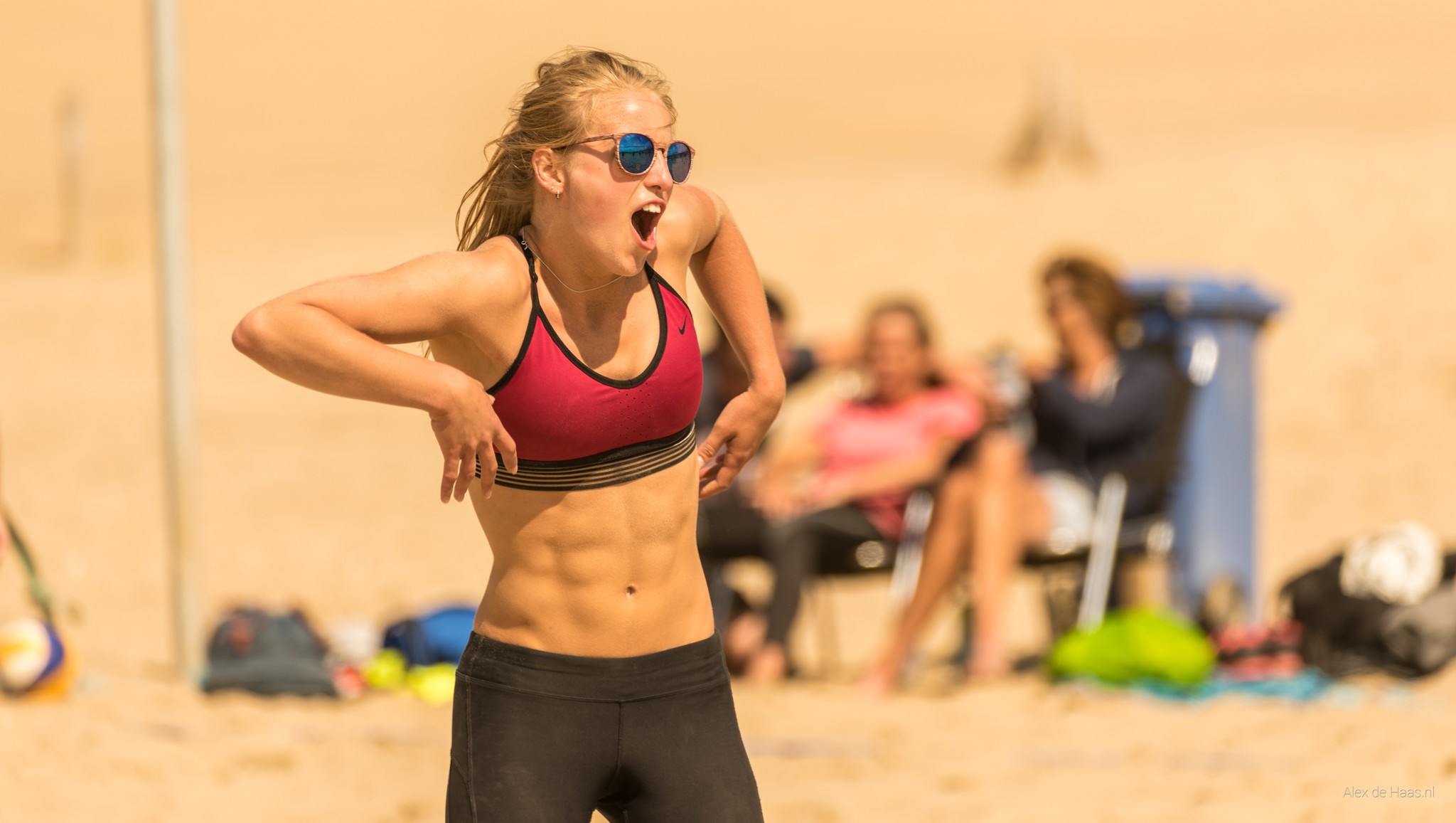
[558,133,694,186]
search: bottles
[996,352,1038,448]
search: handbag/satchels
[196,610,339,698]
[0,620,75,705]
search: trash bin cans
[1122,272,1276,628]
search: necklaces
[521,227,622,293]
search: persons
[231,49,785,823]
[688,292,987,684]
[692,281,855,442]
[860,261,1186,694]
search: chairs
[892,341,1194,631]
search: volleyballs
[0,617,80,704]
